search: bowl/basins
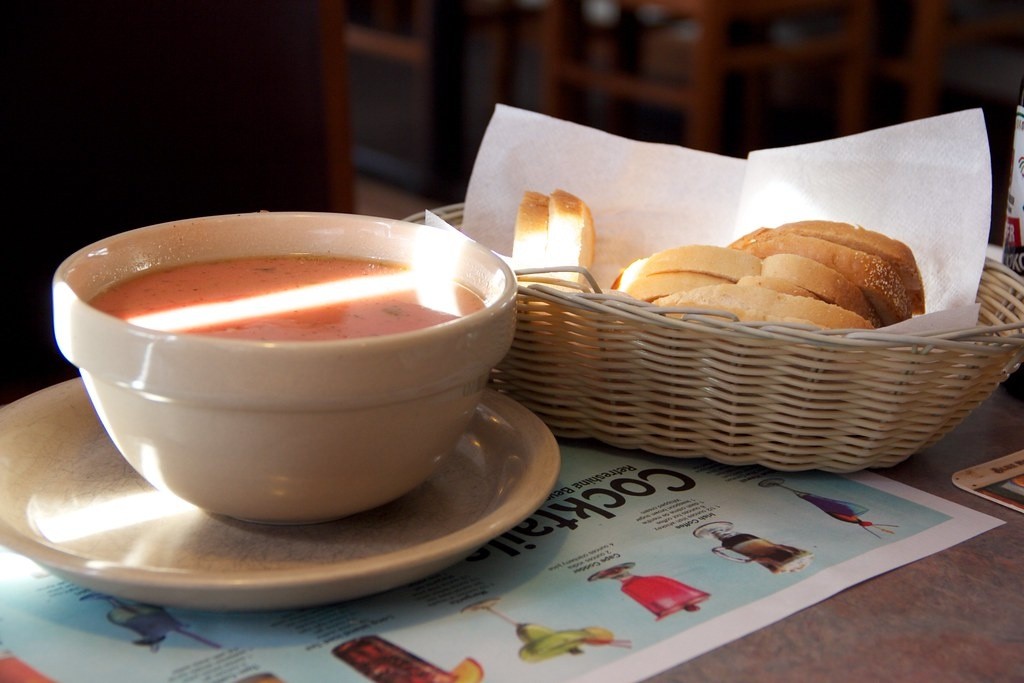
[51,210,519,523]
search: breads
[510,189,926,329]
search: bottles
[1001,77,1023,404]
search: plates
[0,376,562,612]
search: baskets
[403,204,1024,472]
